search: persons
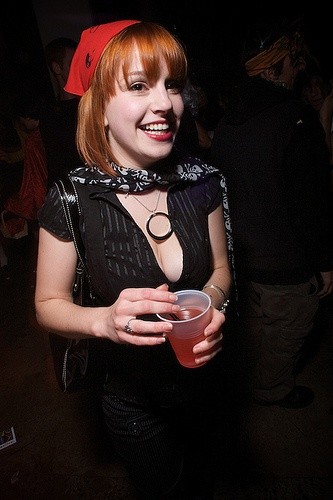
[295,70,333,171]
[207,28,333,408]
[18,39,211,196]
[35,21,237,500]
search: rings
[124,318,137,334]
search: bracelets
[203,285,230,314]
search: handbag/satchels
[48,176,106,393]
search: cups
[155,290,213,368]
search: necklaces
[132,188,173,241]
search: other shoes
[253,385,315,409]
